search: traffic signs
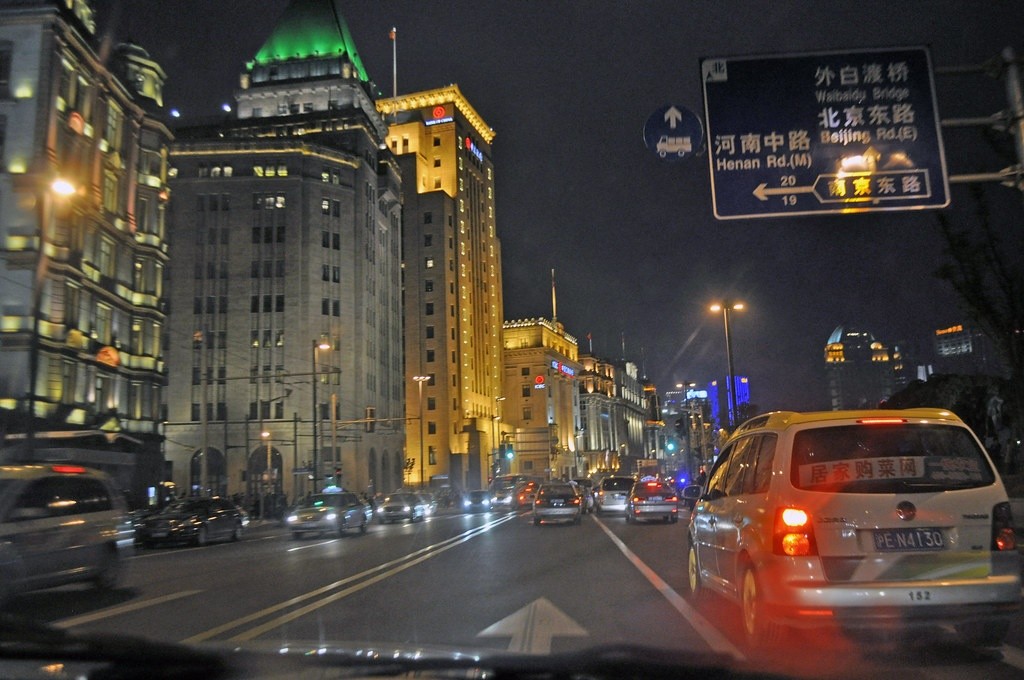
[696,44,952,220]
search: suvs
[680,409,1024,662]
[0,463,129,599]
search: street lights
[261,430,273,494]
[707,297,746,434]
[24,175,75,462]
[312,338,331,497]
[414,376,431,489]
[491,414,500,458]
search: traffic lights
[336,468,343,480]
[674,414,684,440]
[506,443,514,460]
[692,445,704,460]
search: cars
[133,496,244,547]
[286,485,373,539]
[568,478,596,514]
[626,476,680,524]
[376,491,428,524]
[466,490,490,513]
[415,492,438,516]
[532,483,584,525]
[593,476,636,517]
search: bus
[490,474,544,511]
